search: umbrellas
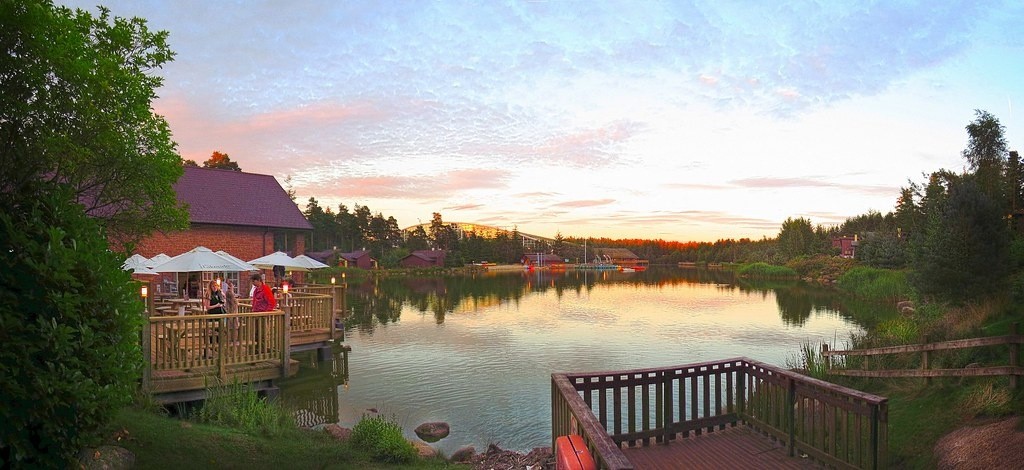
[246,250,314,273]
[275,254,331,268]
[114,246,261,276]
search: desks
[168,298,206,310]
[161,322,247,366]
[277,303,304,325]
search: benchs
[157,305,314,363]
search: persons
[184,274,239,356]
[249,274,276,354]
[273,278,297,308]
